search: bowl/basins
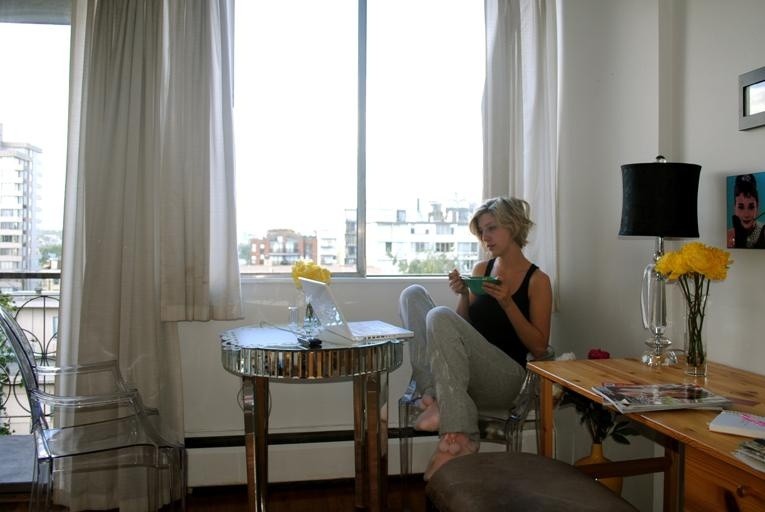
[460,275,502,296]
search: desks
[521,357,765,512]
[221,320,404,512]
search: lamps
[617,155,703,367]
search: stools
[426,452,645,512]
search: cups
[288,306,301,330]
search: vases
[682,296,708,378]
[303,302,320,330]
[573,444,624,495]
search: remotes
[297,334,322,346]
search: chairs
[0,304,190,512]
[398,345,553,487]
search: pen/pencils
[741,414,765,427]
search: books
[731,438,765,474]
[710,410,765,440]
[591,383,732,414]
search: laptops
[297,275,417,342]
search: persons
[727,174,764,249]
[400,195,553,482]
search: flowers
[289,261,330,318]
[562,348,642,444]
[652,241,732,364]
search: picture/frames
[738,66,765,131]
